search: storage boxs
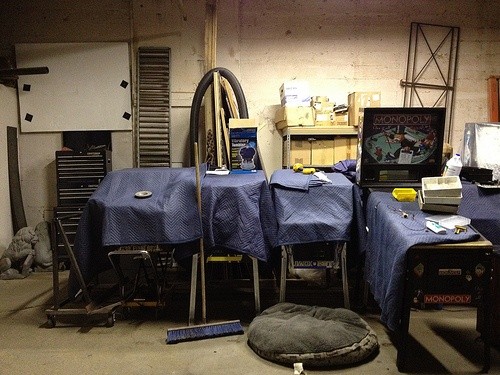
[416,175,464,214]
[275,81,383,169]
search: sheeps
[0,227,39,280]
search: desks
[68,163,500,372]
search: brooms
[162,143,243,343]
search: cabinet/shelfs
[282,126,362,170]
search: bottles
[441,153,463,176]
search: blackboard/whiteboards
[15,41,133,134]
